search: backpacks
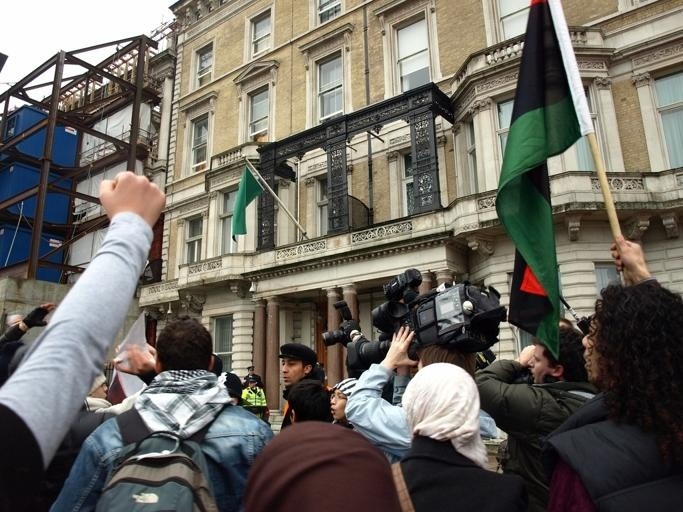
[91,404,231,512]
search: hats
[247,366,254,370]
[248,378,256,382]
[334,378,357,399]
[278,343,317,367]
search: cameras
[321,300,361,347]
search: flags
[229,167,264,244]
[492,1,597,361]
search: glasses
[329,393,347,401]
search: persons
[1,169,168,510]
[0,233,683,510]
